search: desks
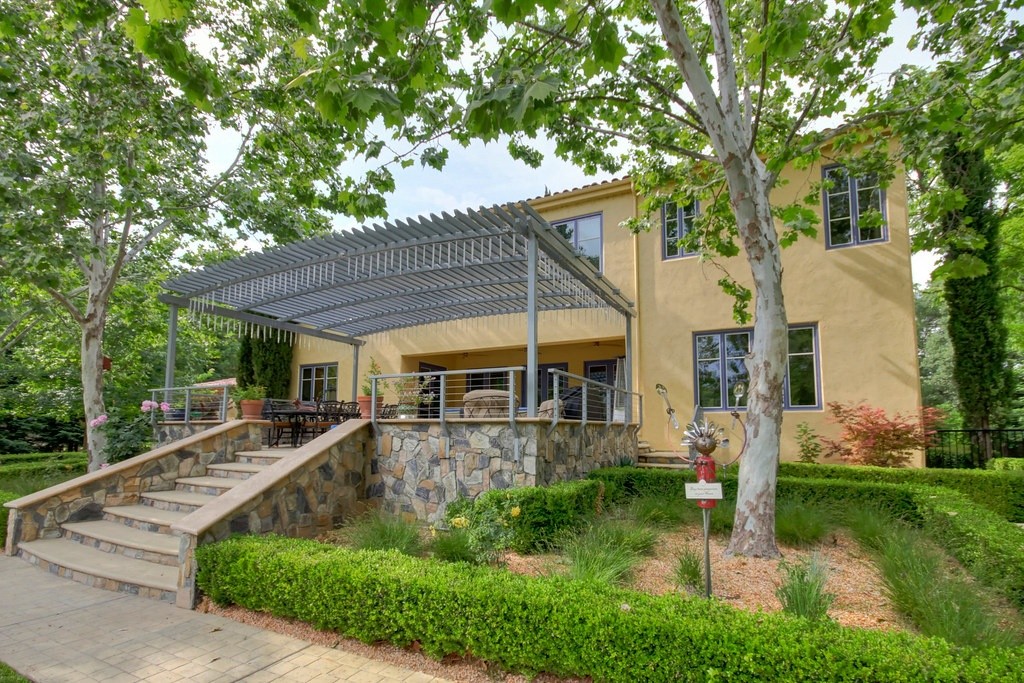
[266,410,361,447]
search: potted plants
[235,382,267,420]
[390,371,435,418]
[358,355,388,419]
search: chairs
[381,404,398,419]
[463,389,520,418]
[265,398,361,449]
[537,399,567,417]
